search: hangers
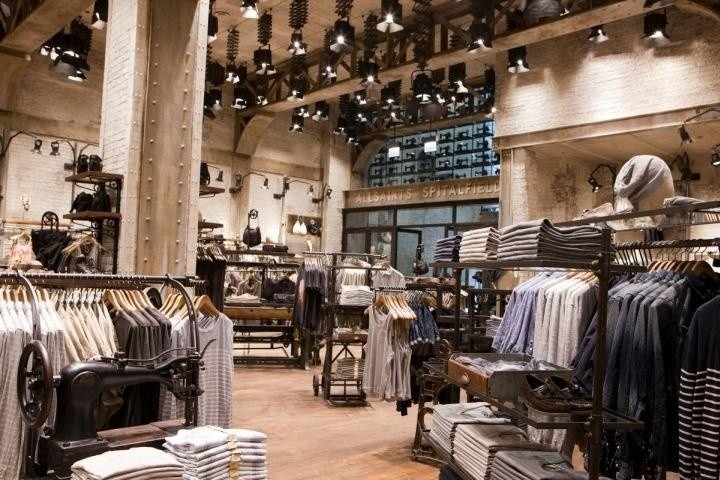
[301,249,391,273]
[0,271,219,322]
[61,229,111,257]
[567,267,601,288]
[240,271,261,286]
[364,285,438,322]
[10,227,30,242]
[613,236,720,281]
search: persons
[472,271,496,307]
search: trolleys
[312,253,373,408]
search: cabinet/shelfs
[221,237,305,369]
[409,228,645,479]
[197,182,227,314]
[65,169,124,274]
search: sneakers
[572,203,614,220]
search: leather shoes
[545,370,593,416]
[518,373,572,418]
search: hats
[614,155,670,202]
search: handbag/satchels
[89,155,102,171]
[200,161,210,185]
[242,225,261,246]
[90,191,111,210]
[77,154,89,173]
[70,192,94,213]
[412,259,428,275]
[30,229,68,254]
[292,221,309,235]
